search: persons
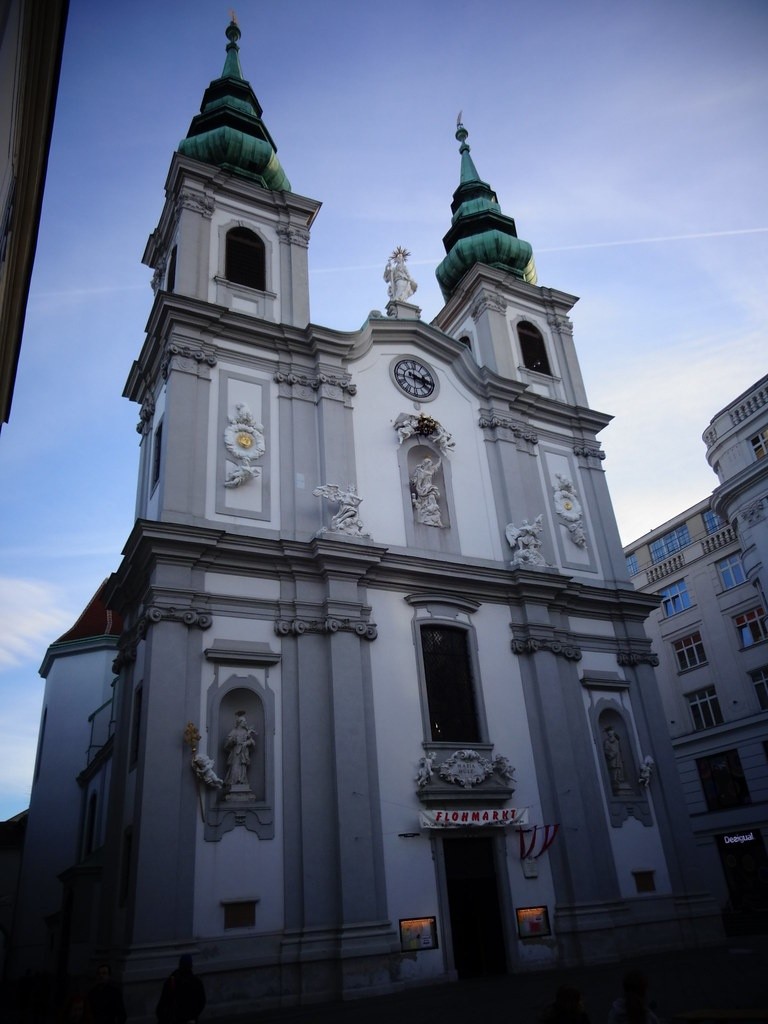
[492,753,516,782]
[82,964,126,1024]
[155,951,206,1024]
[384,246,417,301]
[505,514,542,553]
[225,457,261,489]
[608,970,658,1024]
[574,519,589,546]
[638,754,657,788]
[223,716,255,797]
[314,482,362,524]
[196,754,223,788]
[411,456,443,526]
[540,984,589,1024]
[414,751,437,787]
[605,729,628,784]
